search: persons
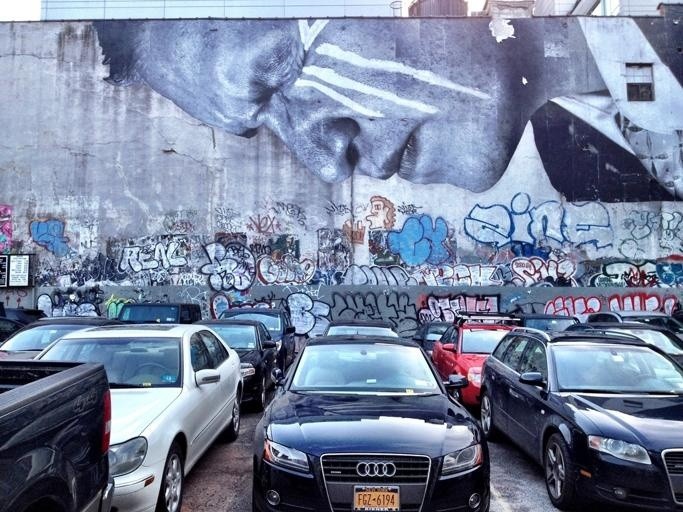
[90,21,683,202]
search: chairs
[88,346,179,387]
[305,351,346,388]
